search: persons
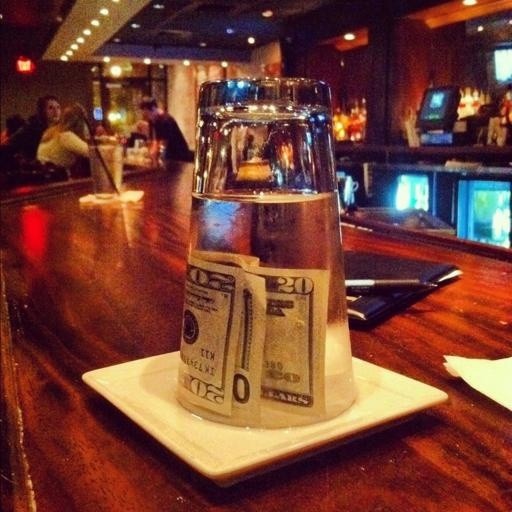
[0,93,190,187]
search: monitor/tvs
[488,43,512,90]
[414,86,461,129]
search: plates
[80,190,145,204]
[83,347,449,490]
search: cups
[175,76,358,430]
[86,136,125,200]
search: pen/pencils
[344,279,438,295]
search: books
[344,249,465,322]
